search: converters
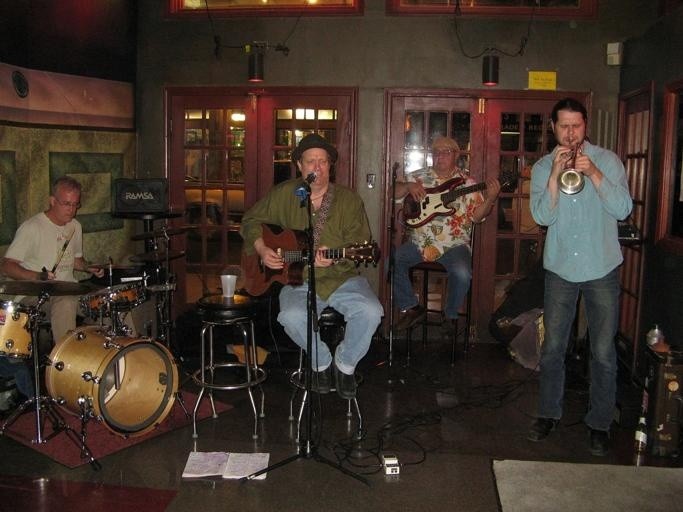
[382,453,401,475]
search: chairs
[396,191,485,367]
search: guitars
[402,170,522,228]
[239,224,379,296]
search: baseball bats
[633,377,650,452]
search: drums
[45,323,180,438]
[0,299,40,359]
[82,281,147,317]
[144,270,178,293]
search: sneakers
[332,352,358,400]
[397,306,425,329]
[588,429,609,456]
[313,366,331,394]
[527,419,559,442]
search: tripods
[238,186,372,488]
[363,176,424,382]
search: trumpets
[559,140,586,194]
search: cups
[223,298,235,307]
[220,274,238,297]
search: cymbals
[131,227,194,242]
[1,279,89,296]
[128,250,185,262]
[89,264,136,271]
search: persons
[2,175,105,348]
[238,133,384,400]
[524,95,634,455]
[387,136,502,331]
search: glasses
[433,149,452,155]
[52,194,81,209]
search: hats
[291,134,337,165]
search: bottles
[635,408,648,454]
[635,455,646,467]
[646,322,666,347]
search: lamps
[203,2,309,84]
[453,0,538,87]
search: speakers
[113,177,168,214]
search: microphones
[392,160,401,175]
[298,170,318,192]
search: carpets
[493,457,681,510]
[0,386,234,511]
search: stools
[191,292,365,441]
